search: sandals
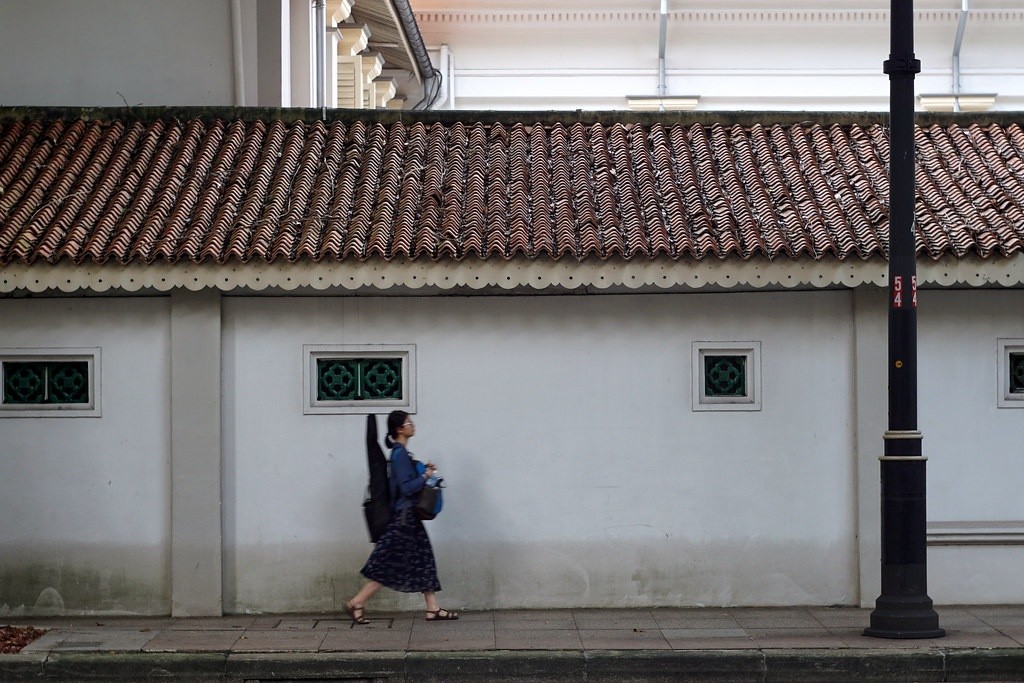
[426,608,459,621]
[343,600,371,624]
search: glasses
[401,420,413,427]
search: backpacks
[393,448,446,520]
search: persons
[345,410,459,624]
